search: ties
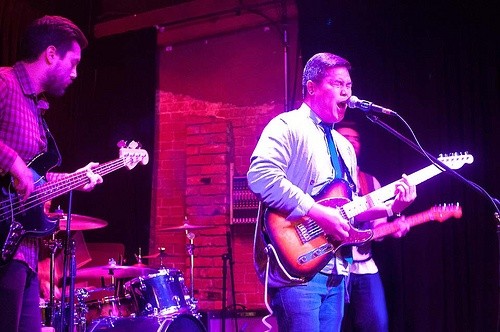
[319,121,353,265]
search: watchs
[384,201,393,217]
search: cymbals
[69,265,160,279]
[158,224,218,233]
[141,254,186,258]
[59,213,107,231]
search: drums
[65,287,138,326]
[125,270,198,316]
[85,314,209,332]
[39,300,63,327]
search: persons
[0,16,103,331]
[247,53,417,331]
[334,121,409,331]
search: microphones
[346,95,397,116]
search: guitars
[0,140,149,269]
[334,202,463,262]
[263,151,475,279]
